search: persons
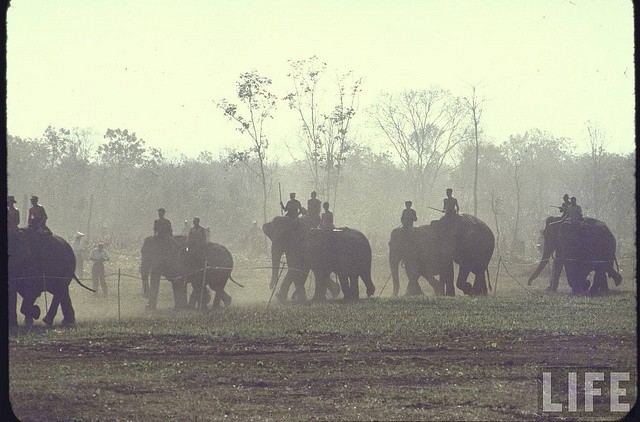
[400,200,417,231]
[280,193,302,219]
[27,194,52,235]
[88,242,109,295]
[71,233,86,281]
[442,187,459,217]
[250,221,260,235]
[189,216,207,244]
[307,191,321,217]
[5,195,21,231]
[559,194,570,220]
[562,197,583,224]
[319,202,333,231]
[152,208,173,237]
[179,220,191,237]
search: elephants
[262,215,339,302]
[430,213,492,296]
[168,235,245,310]
[388,224,456,297]
[527,215,623,296]
[291,216,376,303]
[6,228,98,328]
[139,235,192,309]
